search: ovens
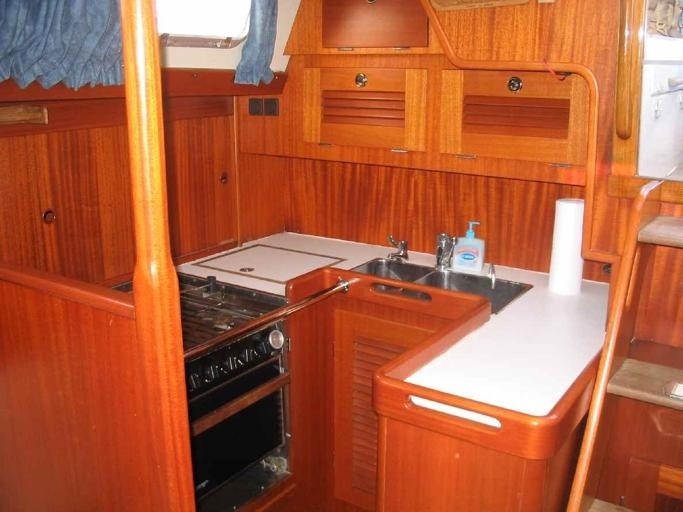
[186,322,287,512]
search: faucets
[436,232,457,270]
[386,232,409,264]
[488,261,496,290]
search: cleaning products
[452,219,486,273]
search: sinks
[346,257,438,295]
[397,269,534,314]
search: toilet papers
[547,198,584,297]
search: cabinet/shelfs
[0,0,682,512]
